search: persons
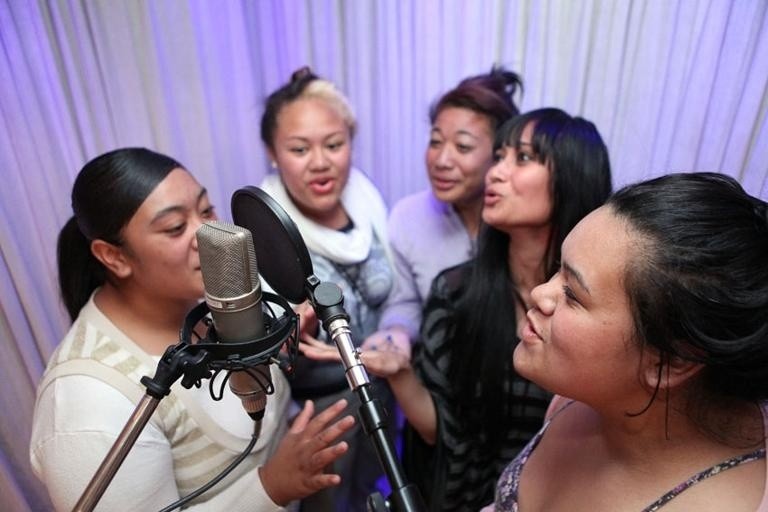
[296,61,524,511]
[250,63,389,509]
[29,146,354,509]
[481,170,766,512]
[356,106,613,512]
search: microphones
[195,220,272,421]
